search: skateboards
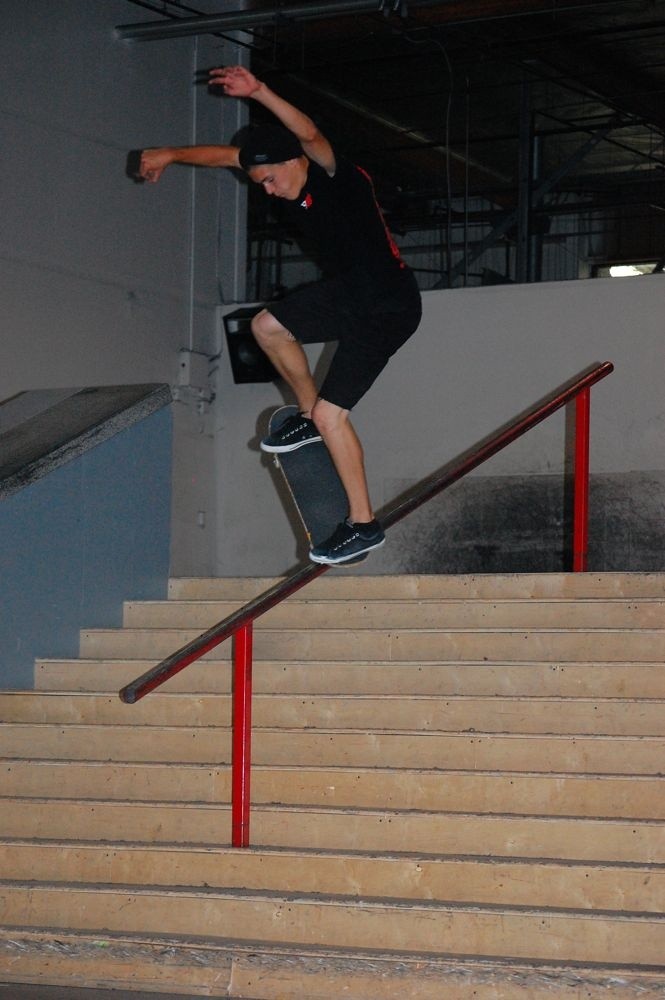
[266,403,370,569]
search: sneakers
[309,518,385,564]
[260,412,323,452]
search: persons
[138,64,424,563]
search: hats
[239,121,307,167]
